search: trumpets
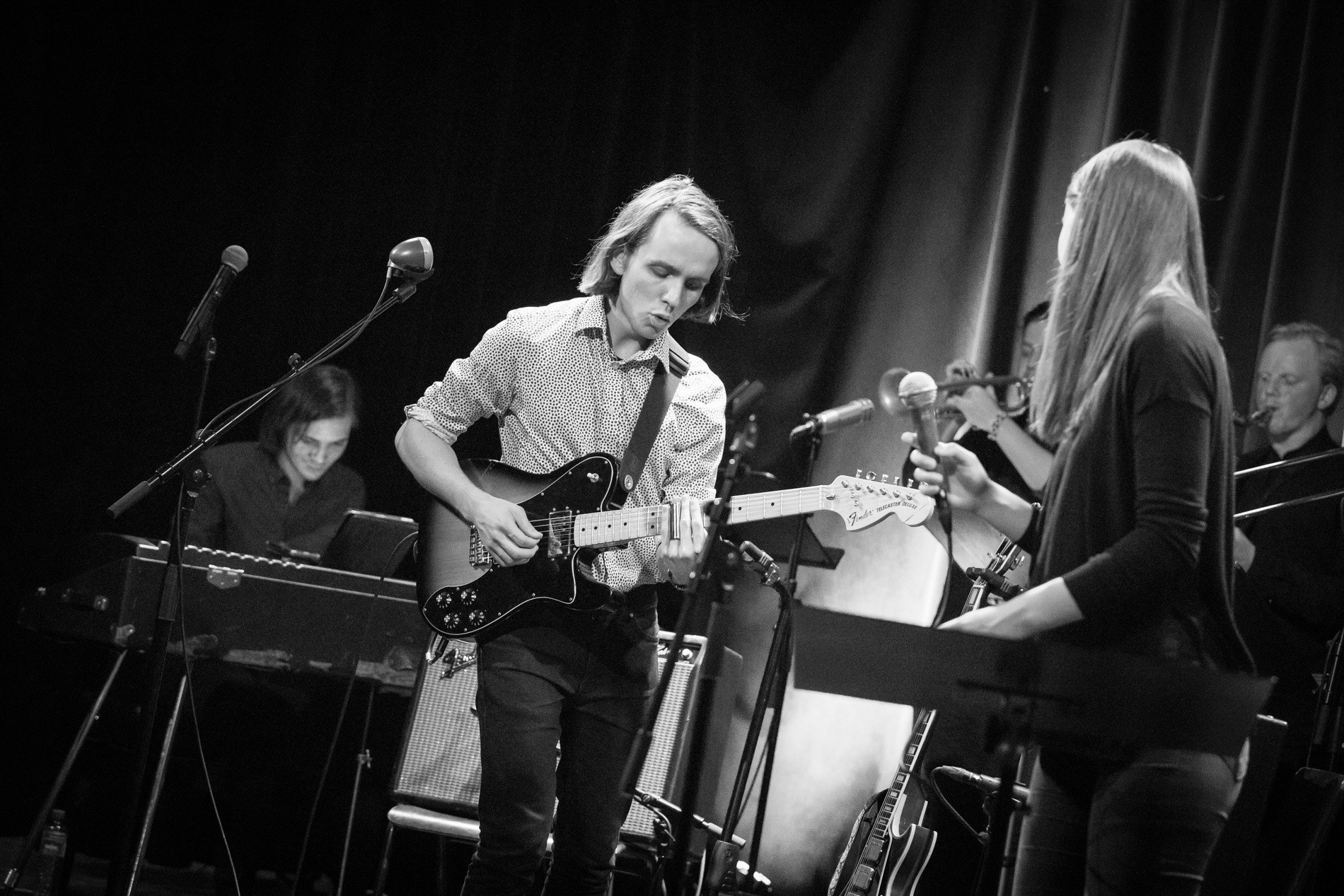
[877,368,1035,424]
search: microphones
[791,398,876,437]
[941,764,1030,808]
[387,237,434,279]
[174,244,248,362]
[898,372,952,533]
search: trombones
[1230,407,1344,525]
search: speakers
[390,629,741,863]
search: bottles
[29,809,67,896]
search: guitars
[415,449,941,644]
[826,532,1024,896]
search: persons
[170,364,369,570]
[938,138,1270,896]
[395,170,734,896]
[1229,321,1344,789]
[898,300,1058,896]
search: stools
[376,803,627,896]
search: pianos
[2,530,429,895]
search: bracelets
[987,410,1006,441]
